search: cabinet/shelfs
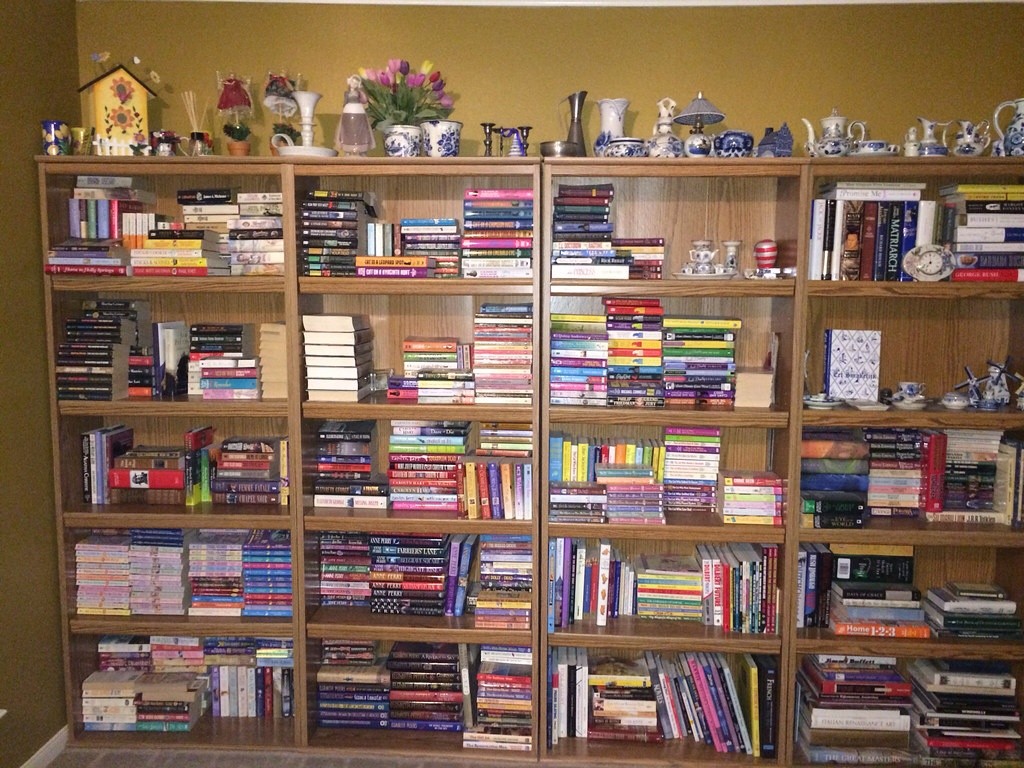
[31,151,1024,768]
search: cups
[178,131,213,156]
[419,119,463,157]
[680,262,733,273]
[857,141,890,154]
[891,381,927,403]
[40,121,92,155]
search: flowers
[357,58,455,133]
[123,56,141,66]
[91,50,113,73]
[273,123,301,143]
[143,70,160,87]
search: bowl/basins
[604,137,649,158]
[540,140,578,157]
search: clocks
[902,244,956,282]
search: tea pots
[801,108,866,157]
[689,245,719,263]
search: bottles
[977,390,1000,411]
[593,98,686,158]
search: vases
[419,119,464,157]
[593,98,630,157]
[39,119,71,156]
[381,124,421,158]
[277,89,339,158]
[269,140,289,156]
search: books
[81,635,294,731]
[56,299,289,398]
[795,654,1024,768]
[800,428,1024,528]
[314,420,533,520]
[549,298,773,407]
[317,639,532,752]
[320,531,533,629]
[75,528,292,616]
[548,538,780,634]
[303,535,321,607]
[797,544,1024,639]
[808,182,1024,282]
[307,641,322,723]
[81,424,290,504]
[302,432,316,507]
[549,427,782,525]
[300,189,533,278]
[547,646,775,758]
[551,184,664,280]
[302,303,533,404]
[824,329,881,401]
[46,175,284,276]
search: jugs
[557,90,588,156]
[903,97,1024,156]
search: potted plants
[222,121,253,157]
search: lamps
[671,90,726,158]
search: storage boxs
[85,127,96,156]
[70,126,90,155]
[734,366,774,409]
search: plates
[902,243,957,282]
[672,273,736,279]
[804,397,928,410]
[847,150,896,157]
